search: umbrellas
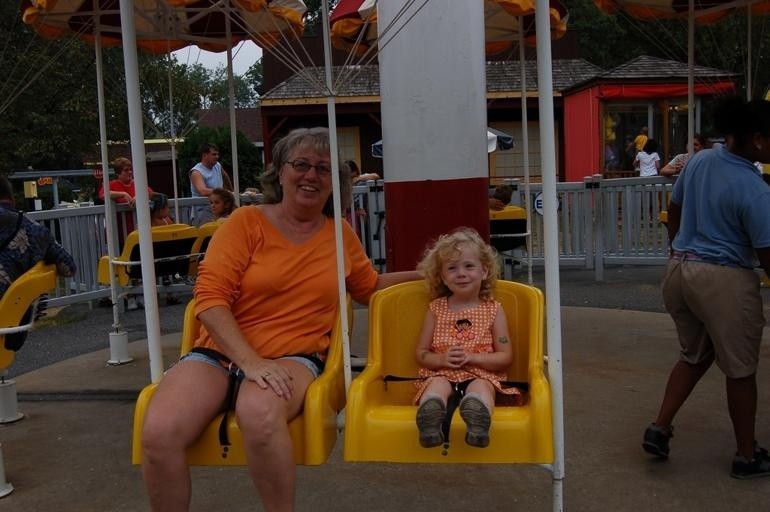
[591,0,769,176]
[372,126,516,160]
[18,0,569,421]
[24,16,240,226]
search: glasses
[282,159,332,176]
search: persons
[0,174,77,339]
[602,121,704,181]
[418,225,516,451]
[141,128,437,512]
[98,141,240,307]
[344,158,379,192]
[640,97,769,480]
[488,184,511,212]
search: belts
[671,249,704,263]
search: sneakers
[459,396,490,447]
[416,397,444,448]
[642,422,674,457]
[730,440,770,479]
